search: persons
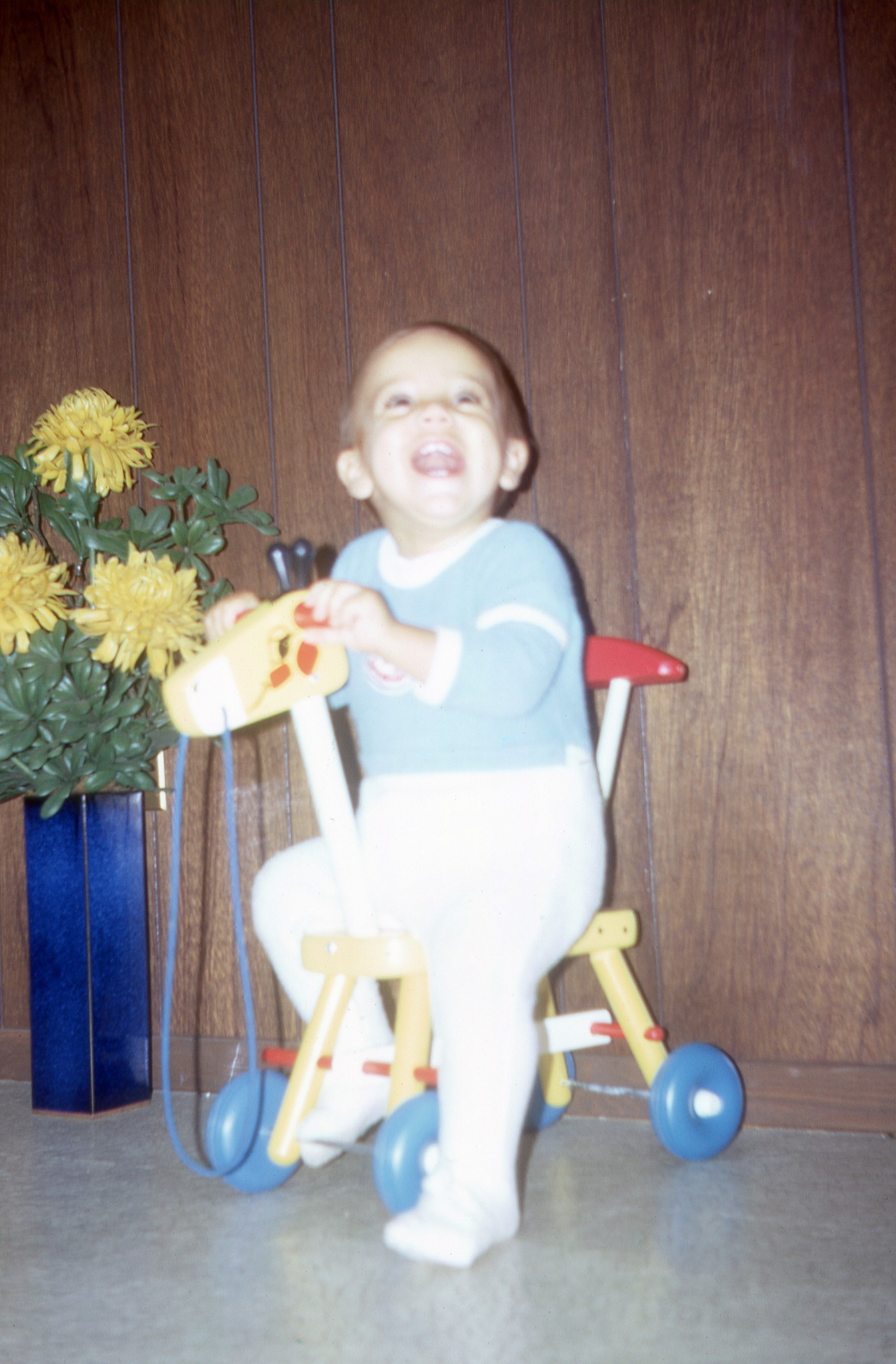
[194,319,617,1278]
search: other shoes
[387,1191,520,1273]
[297,1047,397,1168]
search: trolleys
[163,536,749,1222]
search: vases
[21,792,156,1114]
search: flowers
[0,380,282,823]
